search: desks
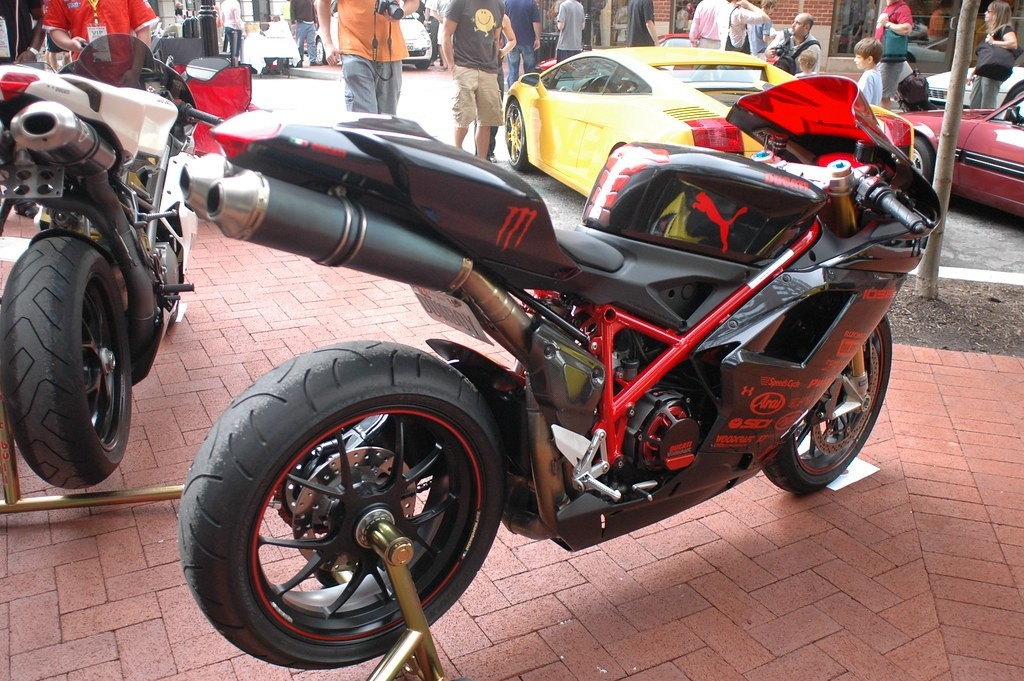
[239,20,301,79]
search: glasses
[988,9,992,13]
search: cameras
[378,0,404,20]
[775,44,786,55]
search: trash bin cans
[534,33,558,63]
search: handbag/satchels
[974,25,1014,82]
[1001,24,1024,60]
[882,3,909,62]
[898,69,937,112]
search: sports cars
[502,45,916,200]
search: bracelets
[885,22,892,29]
[27,46,42,60]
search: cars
[905,38,948,63]
[925,66,1024,110]
[899,89,1024,218]
[314,0,433,71]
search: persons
[0,1,73,76]
[402,0,517,164]
[286,0,324,67]
[965,0,1018,110]
[626,0,659,46]
[551,62,596,93]
[552,0,586,64]
[611,0,628,44]
[316,0,421,119]
[927,0,955,52]
[675,0,822,78]
[215,0,246,68]
[850,1,877,56]
[874,0,913,113]
[42,1,160,90]
[503,0,541,89]
[853,36,882,106]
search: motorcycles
[0,33,225,493]
[171,73,944,673]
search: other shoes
[292,61,302,67]
[489,153,497,163]
[310,62,322,65]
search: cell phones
[81,40,87,46]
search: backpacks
[773,28,821,75]
